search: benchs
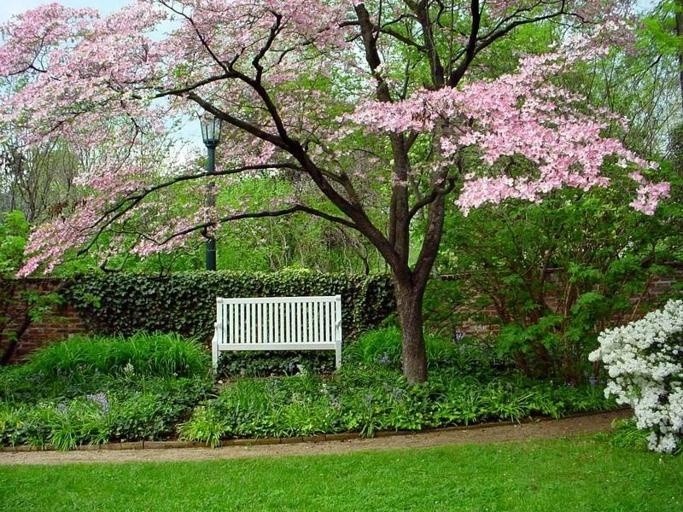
[211,295,342,373]
